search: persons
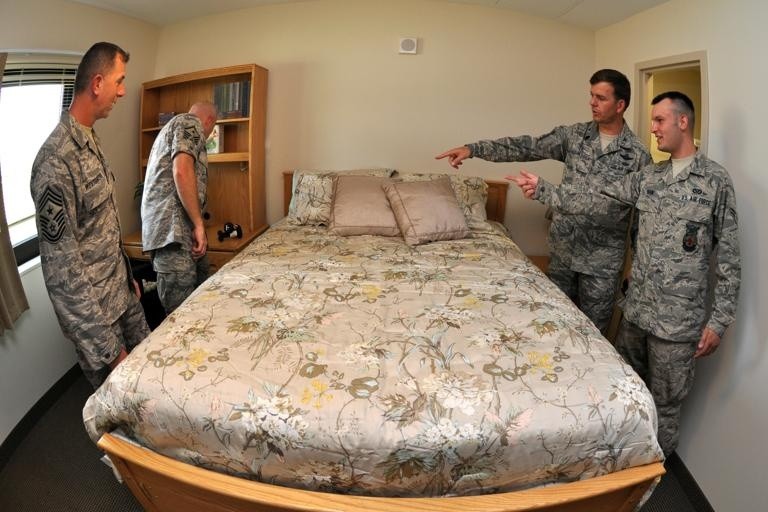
[30,41,151,391]
[434,68,656,339]
[140,100,217,317]
[505,90,742,465]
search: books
[212,80,250,120]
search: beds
[81,170,665,512]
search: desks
[118,62,269,287]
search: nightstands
[527,257,553,274]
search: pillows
[288,170,487,246]
